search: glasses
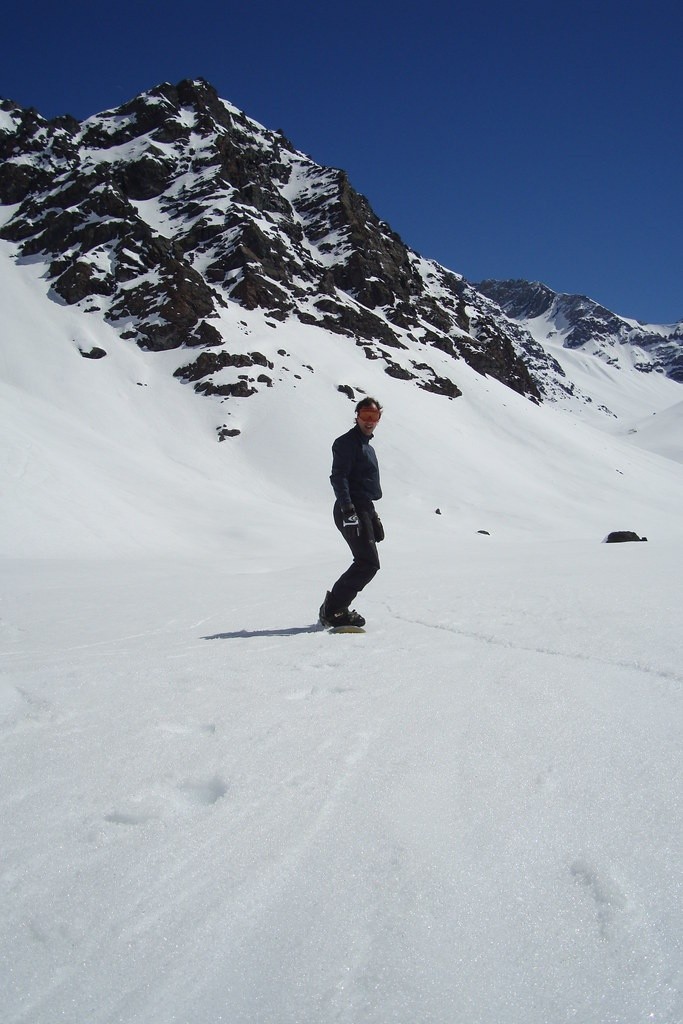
[358,408,380,424]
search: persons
[319,396,385,627]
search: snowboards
[328,626,365,633]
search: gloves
[341,504,359,538]
[372,511,384,542]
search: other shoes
[319,590,365,627]
